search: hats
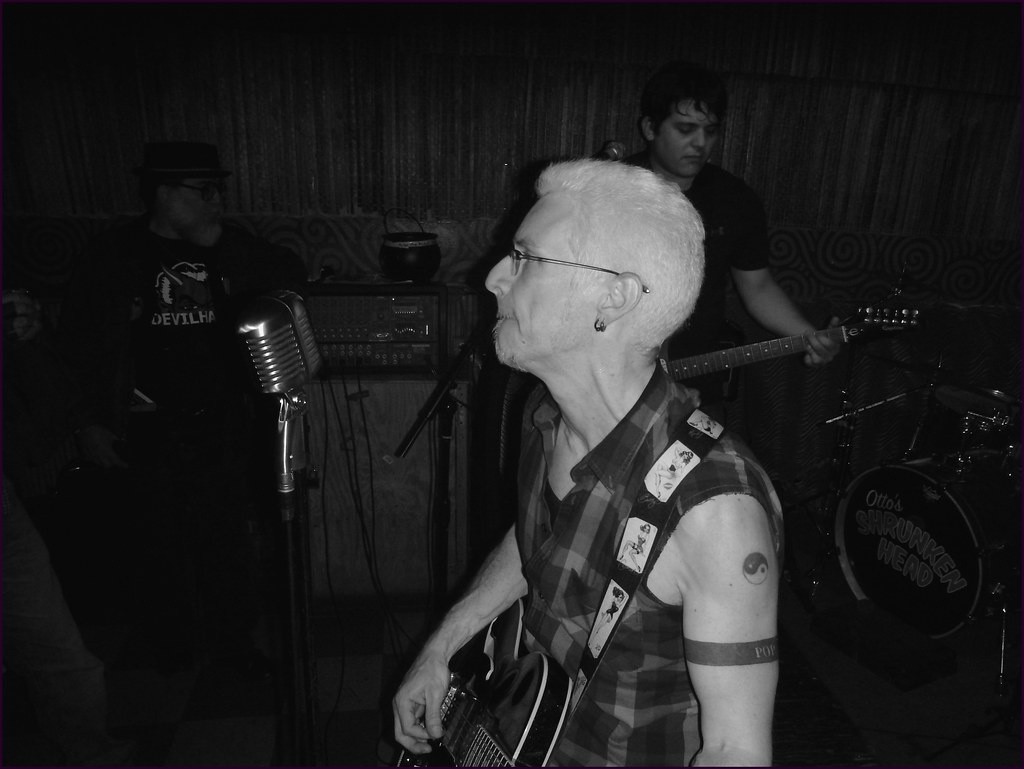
[142,140,233,179]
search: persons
[624,66,842,445]
[1,142,308,767]
[392,159,783,769]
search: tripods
[783,382,936,604]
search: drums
[833,451,1022,641]
[960,413,1018,471]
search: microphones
[590,142,627,163]
[233,288,321,532]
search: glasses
[507,247,650,295]
[162,181,224,201]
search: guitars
[478,303,929,486]
[391,594,577,769]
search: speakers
[291,373,471,612]
